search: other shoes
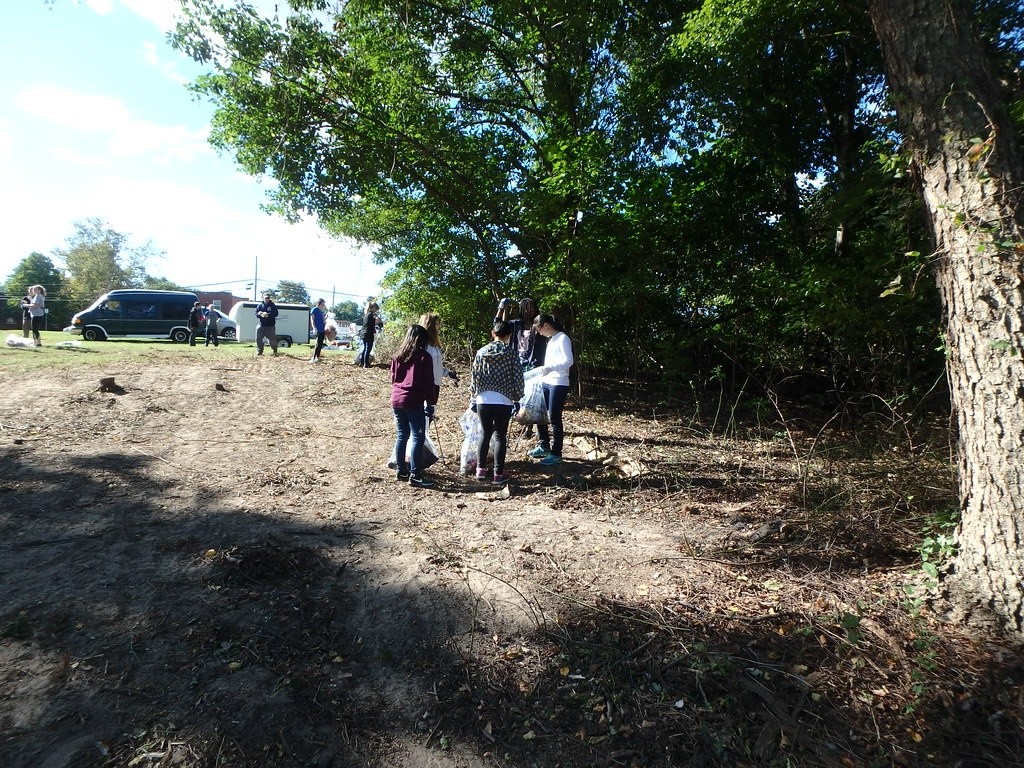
[363,362,373,368]
[258,352,262,355]
[274,351,278,357]
[34,338,41,346]
[313,356,321,362]
[358,362,364,367]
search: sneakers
[521,429,533,439]
[493,469,510,485]
[397,469,410,481]
[409,472,438,489]
[513,427,524,436]
[539,453,563,465]
[476,467,490,479]
[527,444,552,458]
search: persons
[187,301,200,347]
[470,321,525,485]
[21,285,46,347]
[359,302,382,368]
[254,293,279,357]
[204,304,223,347]
[493,298,546,440]
[529,313,573,465]
[310,298,328,363]
[390,313,460,488]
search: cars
[336,330,358,341]
[310,330,317,339]
[201,306,237,340]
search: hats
[263,292,270,299]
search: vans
[70,289,204,343]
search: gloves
[471,402,477,413]
[513,402,520,416]
[424,404,434,421]
[448,370,460,387]
[540,366,552,376]
[258,311,269,318]
[313,328,318,335]
[376,322,383,330]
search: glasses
[537,324,543,333]
